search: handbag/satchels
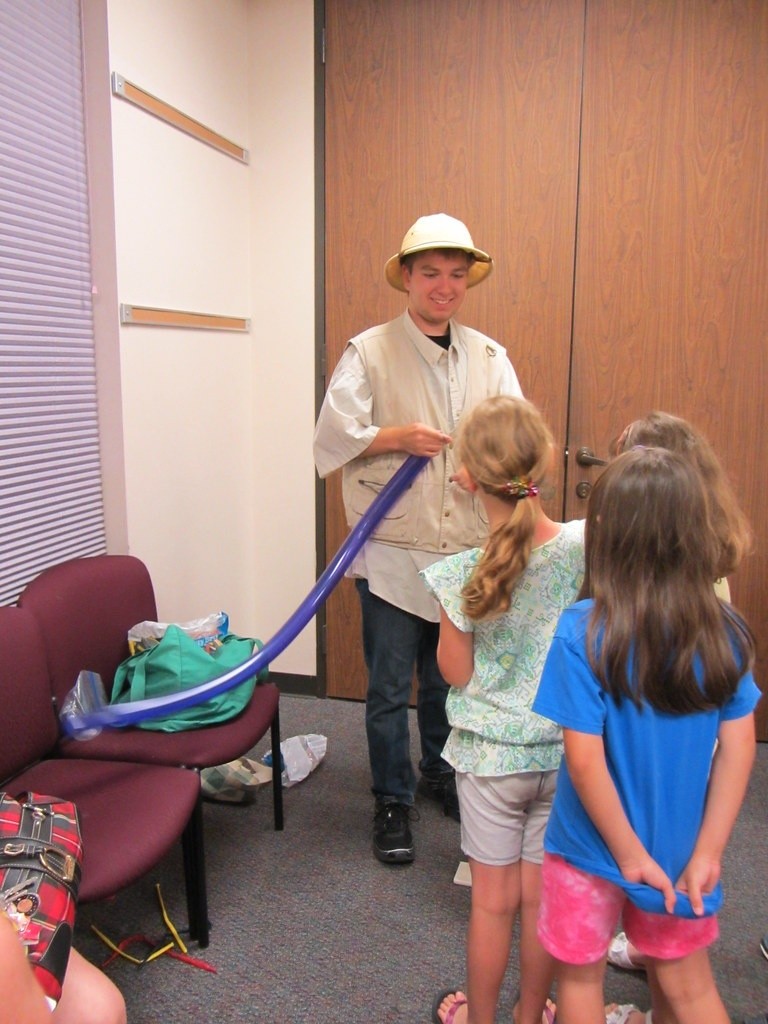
[198,752,273,802]
[263,733,328,787]
[108,611,268,733]
[0,791,85,1007]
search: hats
[385,213,494,293]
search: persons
[605,411,750,968]
[530,447,762,1024]
[419,396,585,1024]
[1,914,127,1024]
[313,213,524,861]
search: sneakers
[420,774,461,821]
[369,796,420,861]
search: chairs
[0,555,284,948]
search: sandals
[432,988,468,1024]
[605,1001,653,1024]
[606,934,649,970]
[540,1004,556,1024]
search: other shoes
[759,939,768,962]
